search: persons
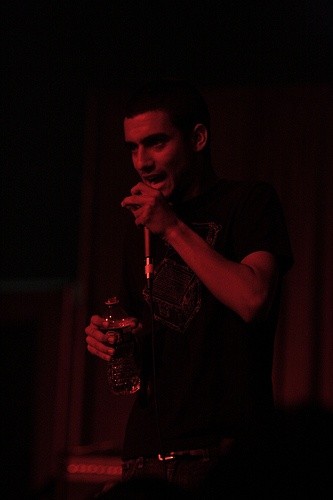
[91,392,333,500]
[83,84,293,481]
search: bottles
[99,296,141,396]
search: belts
[122,445,227,471]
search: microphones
[125,190,154,291]
